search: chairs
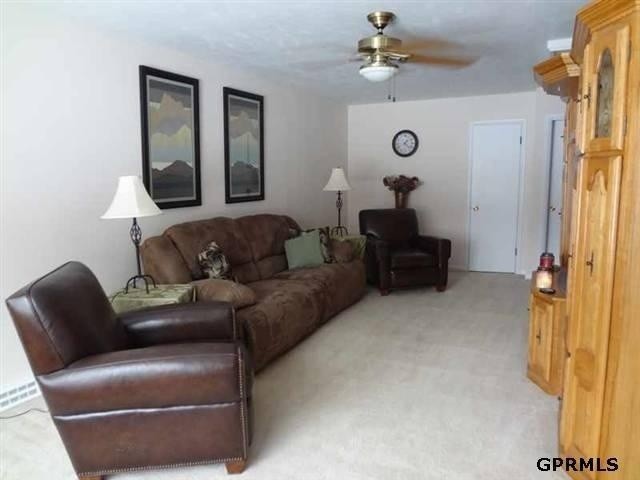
[4,260,253,479]
[358,208,452,297]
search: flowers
[382,175,419,192]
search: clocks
[392,129,419,157]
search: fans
[284,10,480,73]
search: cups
[536,252,558,295]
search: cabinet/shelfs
[525,0,640,479]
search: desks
[330,234,366,260]
[108,283,192,315]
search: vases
[394,191,409,210]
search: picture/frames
[222,86,266,204]
[139,65,203,210]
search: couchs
[139,214,367,376]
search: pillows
[289,226,332,263]
[197,241,237,284]
[283,229,326,271]
[185,278,258,308]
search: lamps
[100,174,163,294]
[358,62,399,84]
[321,168,352,237]
